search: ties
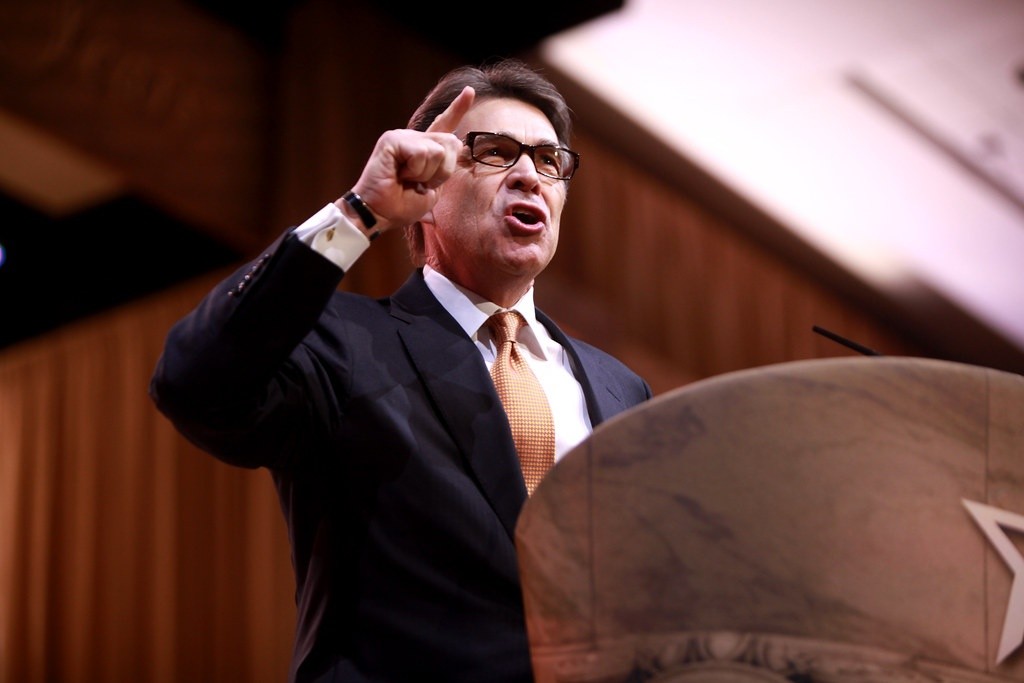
[486,312,556,499]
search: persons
[149,62,654,683]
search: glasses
[461,132,581,180]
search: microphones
[812,325,879,356]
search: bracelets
[338,191,382,248]
